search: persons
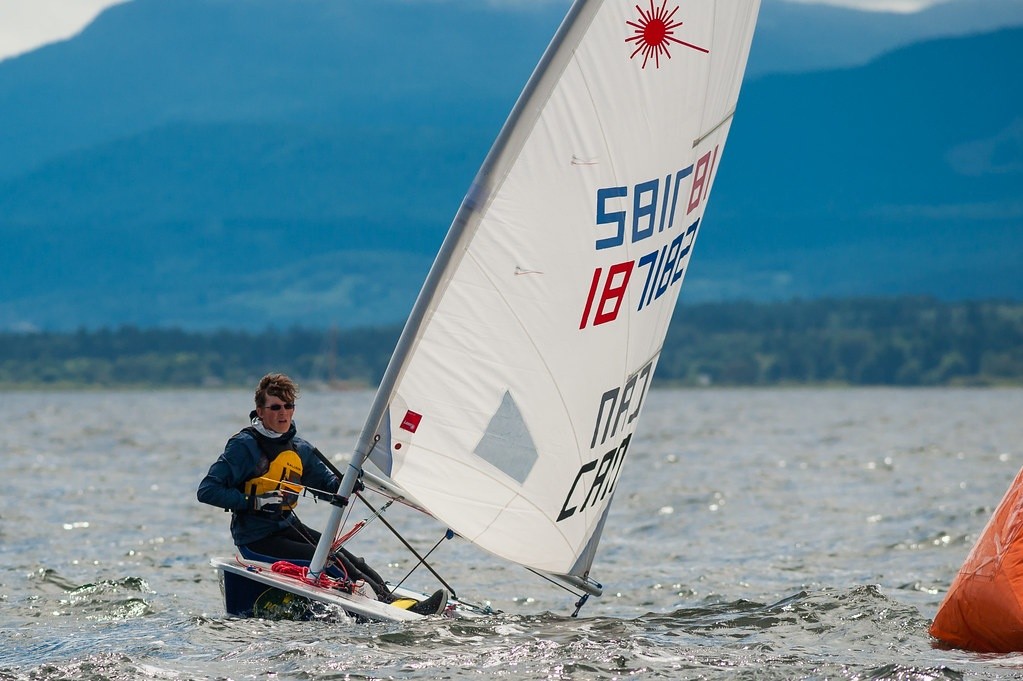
[197,372,448,617]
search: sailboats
[219,0,762,626]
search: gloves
[350,479,365,493]
[256,490,283,512]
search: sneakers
[410,589,448,615]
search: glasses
[264,403,295,411]
